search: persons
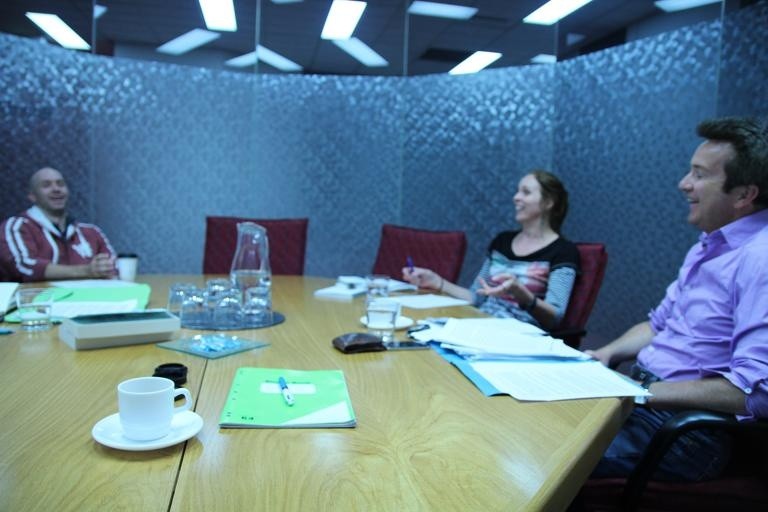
[0,166,118,281]
[400,170,583,334]
[578,116,767,484]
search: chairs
[372,222,467,284]
[558,242,608,351]
[616,413,767,506]
[200,216,311,276]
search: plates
[89,411,203,453]
[357,314,416,331]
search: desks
[1,270,624,512]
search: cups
[363,298,399,343]
[163,281,273,328]
[15,287,52,330]
[115,254,138,282]
[366,274,392,300]
[116,376,190,441]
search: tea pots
[232,221,270,297]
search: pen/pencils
[279,377,294,405]
[408,256,415,274]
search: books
[1,283,22,314]
[0,279,183,351]
[57,308,181,350]
[218,365,358,432]
[314,286,368,300]
